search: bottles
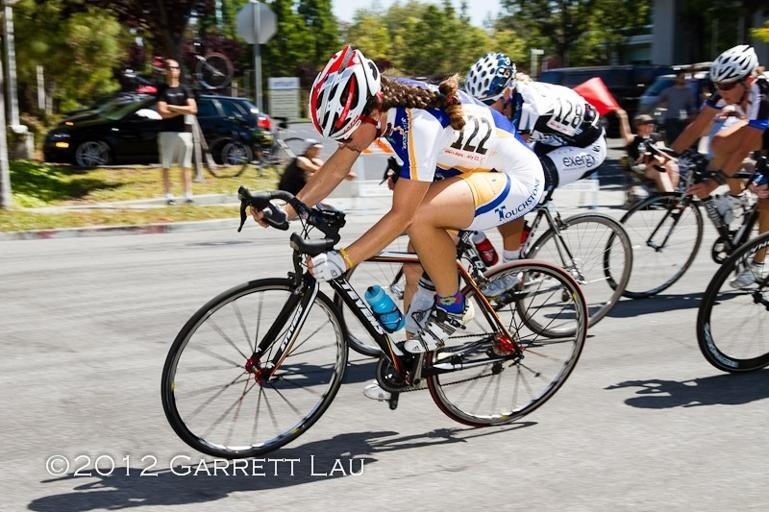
[715,193,734,225]
[364,284,406,332]
[471,231,499,267]
[405,270,438,334]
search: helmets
[710,45,758,83]
[309,46,381,139]
[465,53,516,98]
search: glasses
[481,89,506,105]
[718,81,742,90]
[336,120,362,143]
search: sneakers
[364,384,393,400]
[731,268,769,287]
[715,190,756,215]
[483,270,523,297]
[166,192,192,203]
[404,297,474,353]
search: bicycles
[154,40,236,89]
[383,140,764,379]
[205,113,310,180]
[156,182,589,459]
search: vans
[38,93,277,168]
[539,60,768,133]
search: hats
[635,114,656,124]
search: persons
[465,52,606,298]
[278,138,356,220]
[249,44,545,401]
[157,59,198,202]
[616,44,769,287]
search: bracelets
[339,249,354,269]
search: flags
[572,77,620,117]
